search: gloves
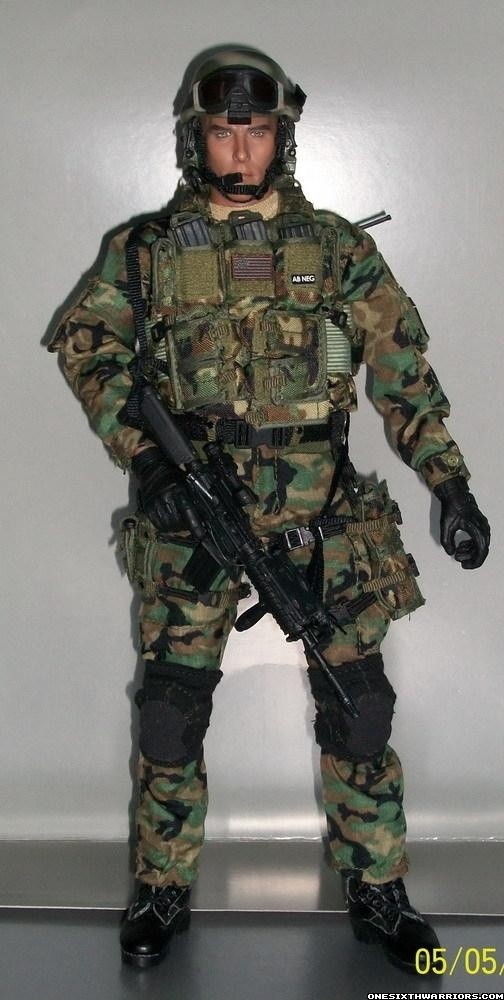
[128,446,199,533]
[432,476,491,570]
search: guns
[137,386,362,719]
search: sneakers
[340,866,443,972]
[120,882,192,967]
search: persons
[41,41,492,977]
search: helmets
[172,44,306,121]
